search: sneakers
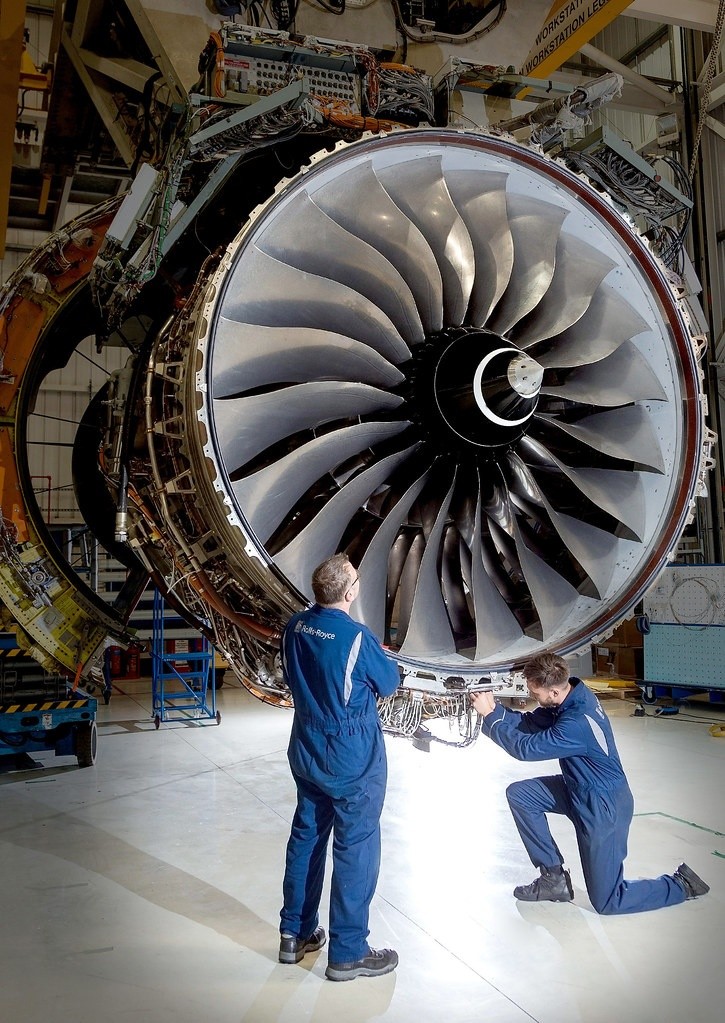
[674,861,712,900]
[325,946,399,982]
[512,865,576,903]
[278,925,327,965]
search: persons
[279,552,400,981]
[471,654,710,916]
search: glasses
[351,567,363,588]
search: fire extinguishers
[111,646,121,674]
[128,642,141,676]
